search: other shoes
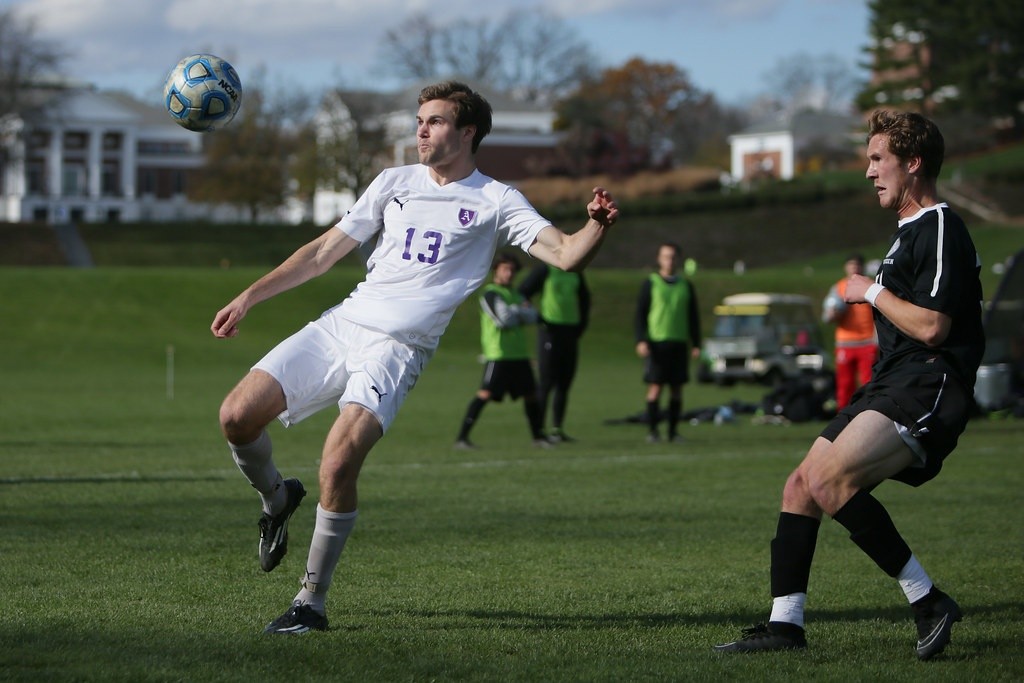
[646,434,687,443]
[538,437,559,447]
[454,440,478,448]
[551,431,576,443]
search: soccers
[163,54,242,133]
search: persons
[714,102,991,665]
[519,231,592,449]
[207,81,618,637]
[457,252,556,445]
[633,238,705,445]
[815,255,879,412]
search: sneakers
[910,584,961,659]
[258,478,306,572]
[711,621,807,653]
[262,604,330,636]
